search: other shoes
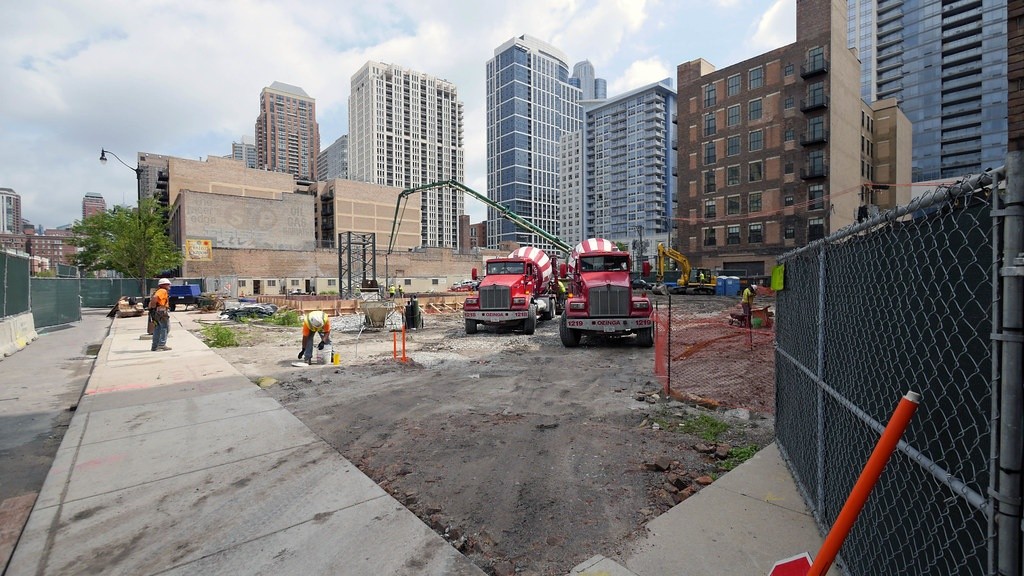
[150,345,171,352]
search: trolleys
[729,312,748,327]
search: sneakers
[304,359,311,365]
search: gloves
[317,341,326,350]
[298,348,305,359]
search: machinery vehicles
[652,242,717,296]
[559,238,655,348]
[462,246,557,335]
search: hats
[751,284,757,292]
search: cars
[451,281,477,290]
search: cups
[334,354,340,365]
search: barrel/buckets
[317,350,331,364]
[753,318,761,328]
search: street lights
[100,147,141,229]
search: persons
[552,279,566,308]
[741,285,760,328]
[695,271,705,288]
[389,284,404,298]
[298,311,330,365]
[355,285,360,294]
[149,278,172,352]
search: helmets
[391,284,394,286]
[156,278,171,285]
[309,314,324,328]
